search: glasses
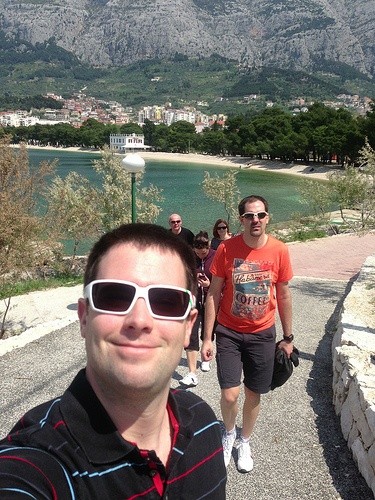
[241,211,267,219]
[82,279,197,320]
[216,226,227,230]
[170,220,181,224]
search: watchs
[283,334,294,341]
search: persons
[0,222,228,500]
[168,214,197,242]
[179,230,215,387]
[201,195,296,471]
[210,220,235,250]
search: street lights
[122,154,145,223]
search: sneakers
[201,361,210,372]
[179,373,197,386]
[236,440,253,472]
[222,429,236,467]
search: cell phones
[199,275,206,282]
